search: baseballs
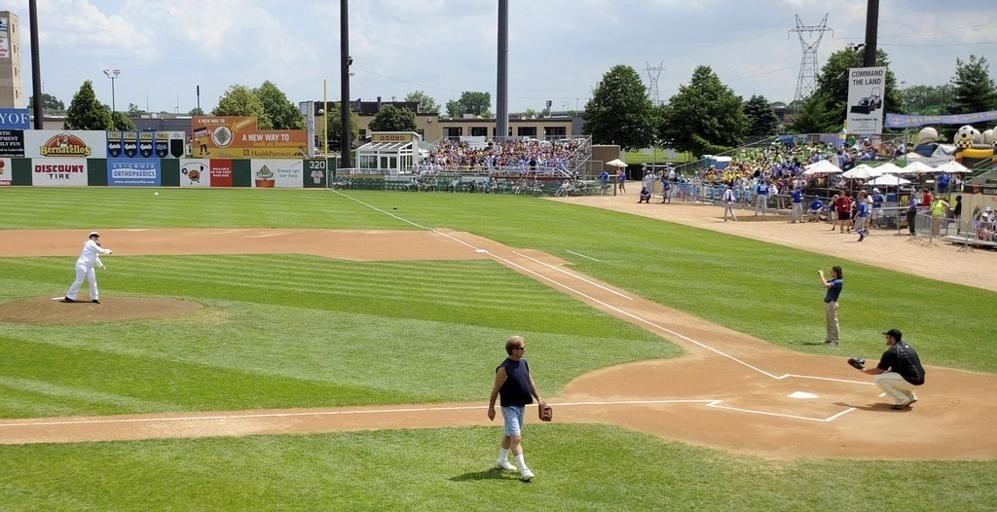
[155,192,159,196]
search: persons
[645,140,962,243]
[618,170,626,195]
[486,336,546,481]
[817,264,844,346]
[848,328,925,411]
[64,231,113,305]
[614,168,620,182]
[411,138,587,178]
[597,168,609,195]
[972,206,996,241]
[638,187,651,203]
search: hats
[873,188,879,192]
[89,232,101,237]
[883,329,901,338]
[982,206,991,217]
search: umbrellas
[603,159,628,169]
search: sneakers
[520,466,534,480]
[497,457,516,472]
[831,227,854,233]
[858,235,863,240]
[891,393,917,410]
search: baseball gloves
[848,357,866,369]
[539,401,552,421]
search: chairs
[332,177,616,196]
